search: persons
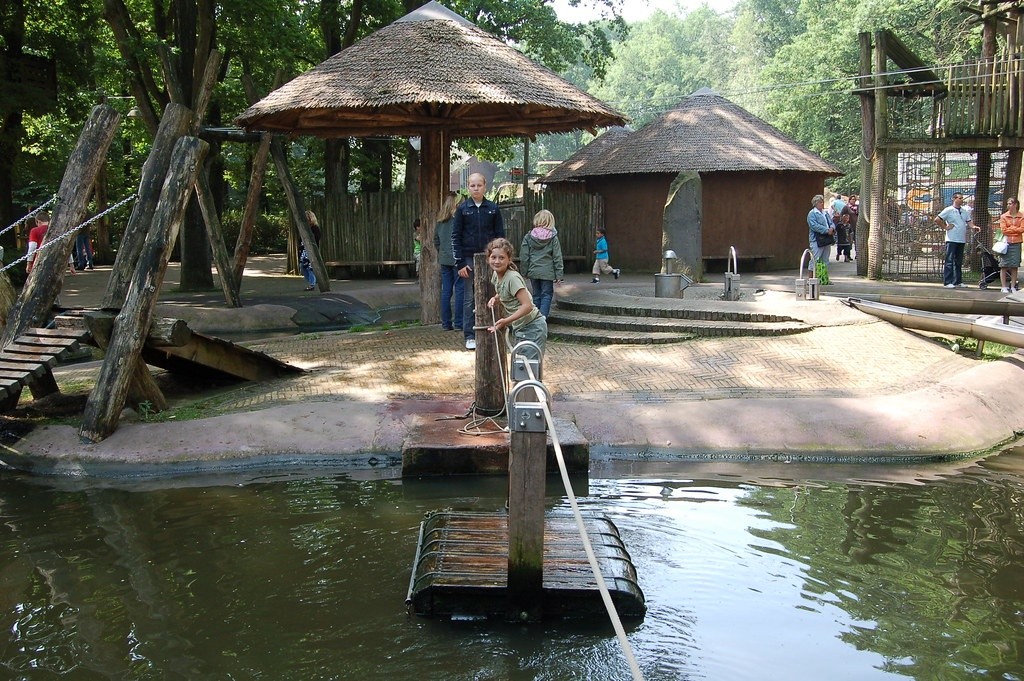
[806,194,836,287]
[413,218,421,280]
[75,207,94,270]
[22,211,77,277]
[590,227,620,283]
[434,192,465,331]
[451,173,505,350]
[933,192,981,289]
[883,196,933,260]
[486,238,548,433]
[86,235,95,268]
[998,198,1024,293]
[840,194,859,259]
[831,194,847,213]
[299,210,321,291]
[519,210,564,317]
[833,211,853,262]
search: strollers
[974,231,1019,290]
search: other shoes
[443,326,454,330]
[1001,287,1009,293]
[304,286,314,291]
[503,426,509,432]
[1010,288,1016,293]
[466,338,476,349]
[454,328,463,331]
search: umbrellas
[234,0,632,328]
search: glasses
[955,197,963,199]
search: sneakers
[958,283,968,288]
[943,284,955,288]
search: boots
[613,269,620,279]
[590,278,600,284]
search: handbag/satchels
[992,241,1007,254]
[817,233,834,247]
